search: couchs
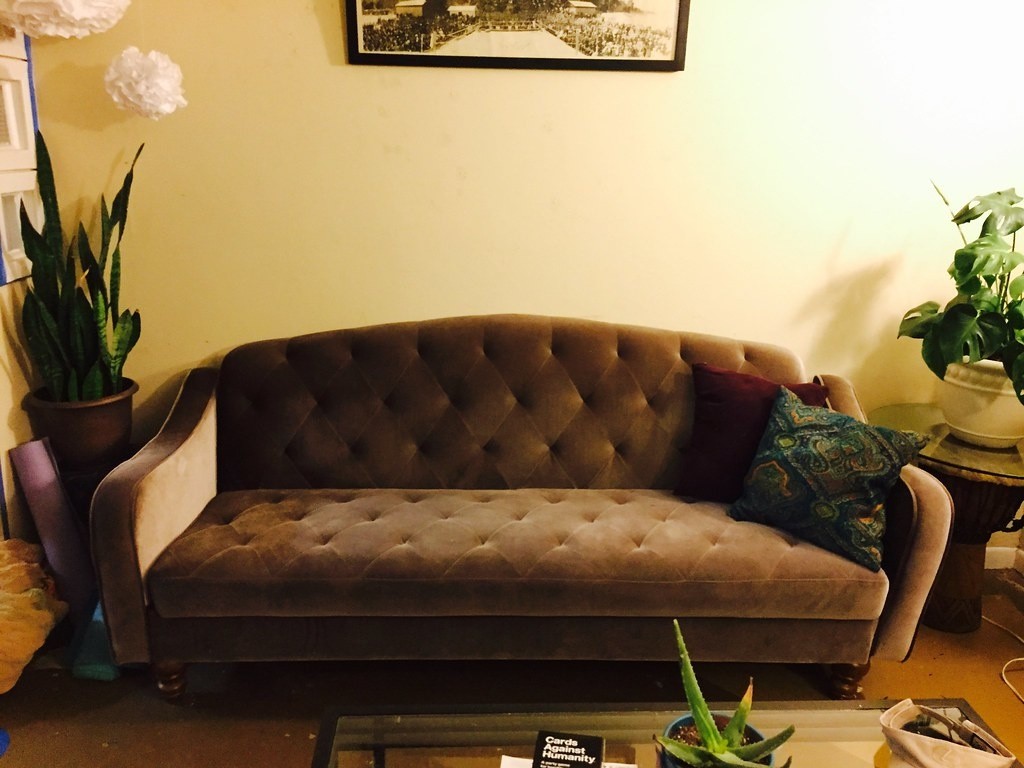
[87,313,955,699]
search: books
[532,728,605,768]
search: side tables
[860,401,1024,635]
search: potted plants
[20,128,147,480]
[652,616,797,768]
[897,179,1024,449]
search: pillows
[671,363,830,505]
[726,385,931,572]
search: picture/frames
[344,0,691,72]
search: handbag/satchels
[878,695,1017,768]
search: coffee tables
[308,696,1024,768]
